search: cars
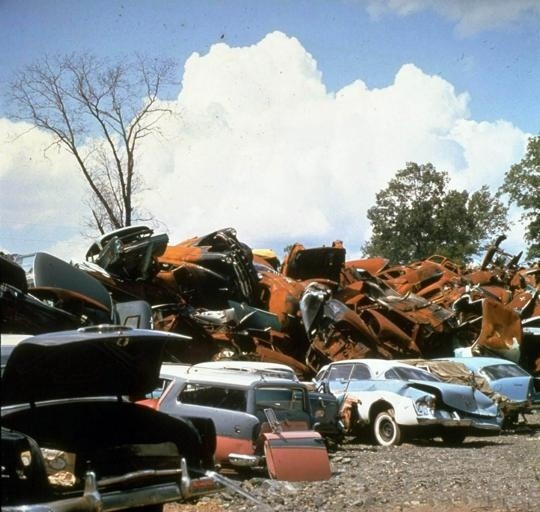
[311,357,505,448]
